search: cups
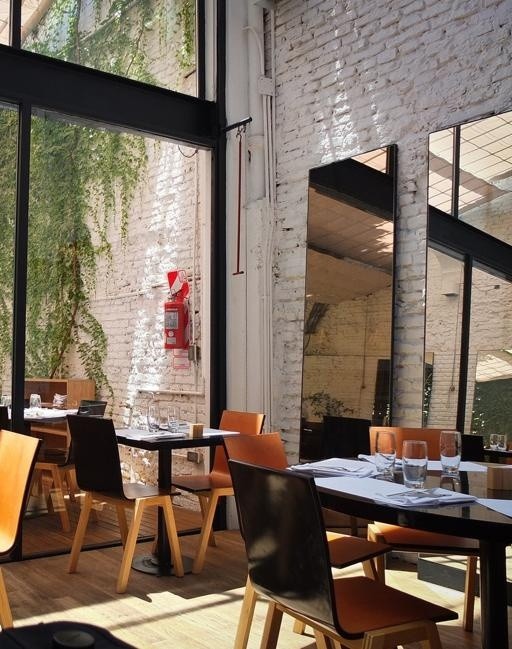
[167,405,180,432]
[375,430,398,478]
[28,393,43,415]
[438,430,463,477]
[401,439,429,490]
[147,404,161,431]
[489,434,508,452]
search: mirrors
[297,143,399,540]
[417,110,512,605]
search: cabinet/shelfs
[24,377,100,454]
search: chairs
[23,397,109,534]
[365,425,481,632]
[151,410,269,576]
[1,428,41,632]
[65,413,186,596]
[223,431,391,648]
[227,455,462,648]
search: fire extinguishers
[164,290,189,349]
